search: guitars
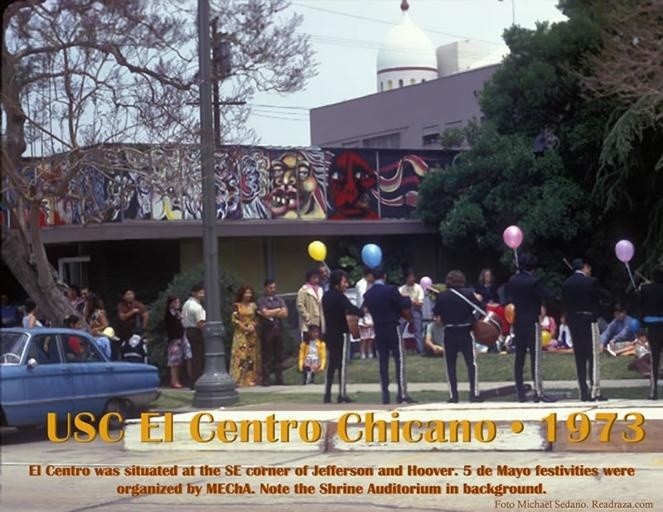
[400,308,416,321]
[425,286,501,346]
[318,260,361,340]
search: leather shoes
[533,395,556,402]
[336,397,352,402]
[590,395,607,401]
[396,397,417,403]
[170,382,184,388]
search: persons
[2,254,663,403]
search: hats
[97,326,120,341]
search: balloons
[307,241,328,262]
[361,243,383,269]
[503,225,524,250]
[615,240,635,264]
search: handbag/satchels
[166,320,184,338]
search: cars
[0,328,161,430]
